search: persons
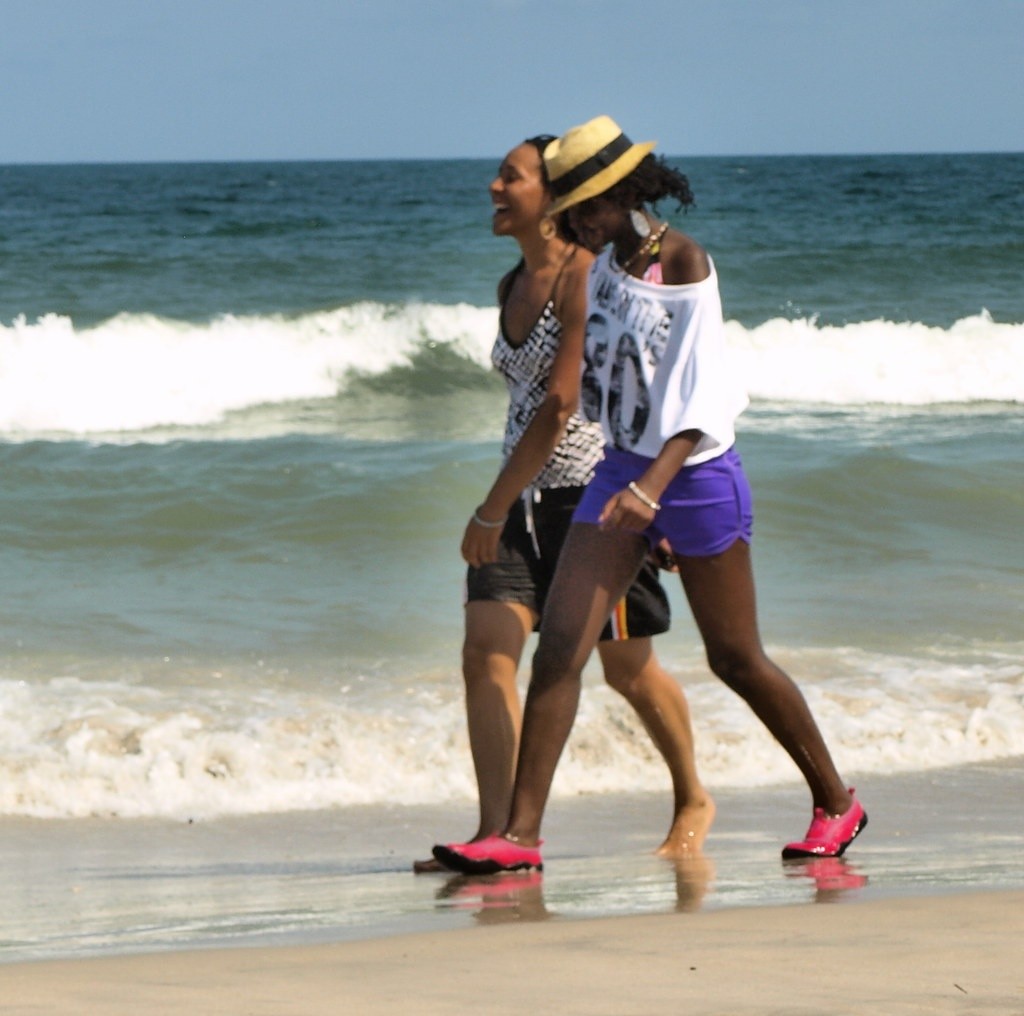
[430,113,868,875]
[414,136,719,872]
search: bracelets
[473,507,507,528]
[629,482,661,510]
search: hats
[543,114,656,216]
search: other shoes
[782,786,867,859]
[432,834,545,873]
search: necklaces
[608,223,667,273]
